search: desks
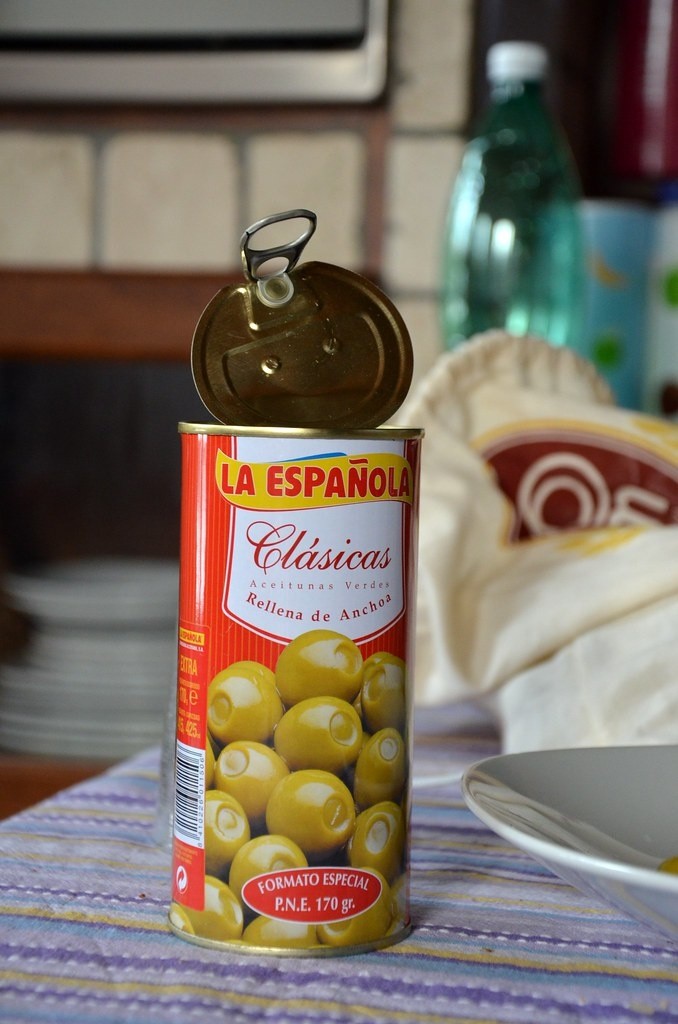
[0,691,678,1024]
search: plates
[460,743,678,944]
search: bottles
[441,41,590,360]
